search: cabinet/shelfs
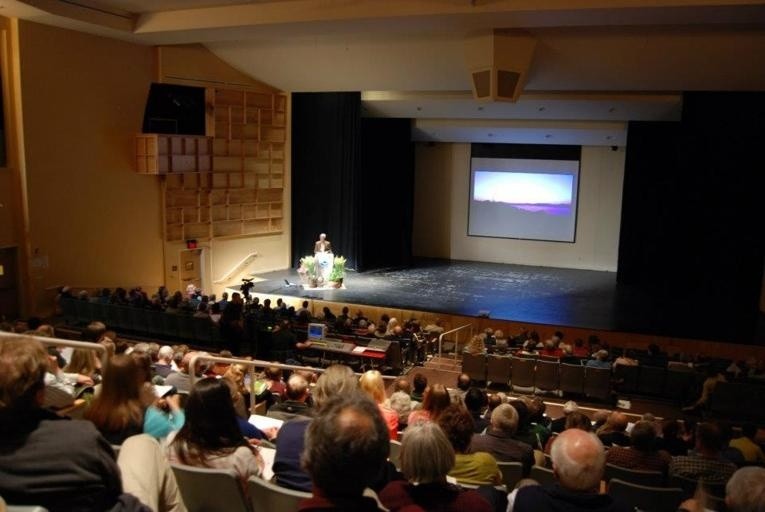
[166,247,204,300]
[135,86,288,241]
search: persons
[2,285,764,512]
[314,233,331,256]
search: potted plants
[299,255,318,288]
[327,254,347,290]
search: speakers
[492,66,528,103]
[470,69,494,102]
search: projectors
[479,310,490,318]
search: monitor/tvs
[307,323,328,340]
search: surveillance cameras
[611,146,619,152]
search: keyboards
[304,339,325,345]
